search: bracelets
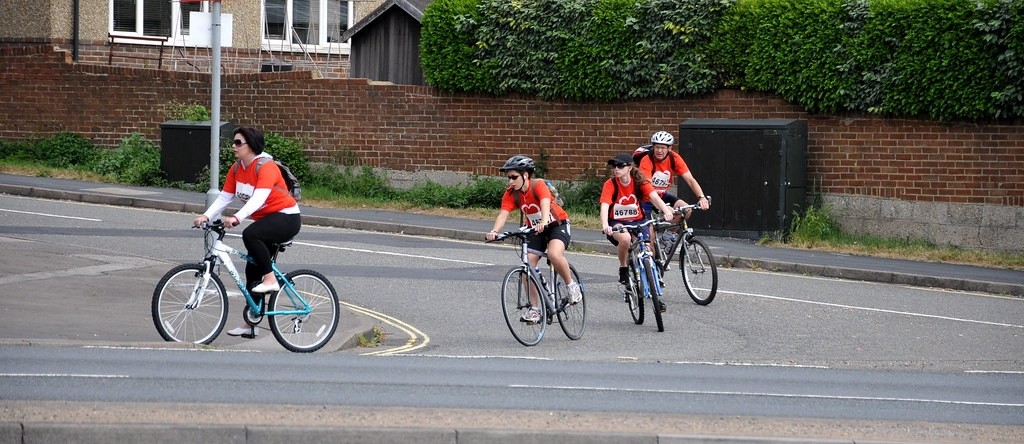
[232,214,241,226]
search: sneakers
[565,282,582,305]
[520,308,542,324]
[659,234,678,255]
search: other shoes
[659,300,666,309]
[252,281,280,292]
[620,272,629,285]
[227,327,259,336]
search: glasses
[230,139,247,148]
[611,164,629,169]
[507,174,520,180]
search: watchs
[698,195,704,199]
[490,230,498,234]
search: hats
[651,131,674,145]
[608,153,633,166]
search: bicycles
[602,215,666,331]
[485,227,587,347]
[654,202,719,306]
[151,219,339,353]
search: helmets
[499,154,537,173]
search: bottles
[664,237,676,254]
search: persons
[485,156,582,322]
[639,131,710,287]
[193,127,301,336]
[599,153,674,310]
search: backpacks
[234,156,302,200]
[632,144,675,174]
[542,177,565,208]
